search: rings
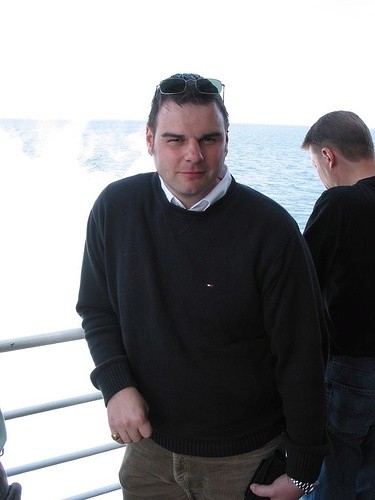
[111,433,121,441]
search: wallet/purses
[245,454,290,500]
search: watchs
[289,476,321,496]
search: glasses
[154,77,226,105]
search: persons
[75,73,329,500]
[301,110,374,500]
